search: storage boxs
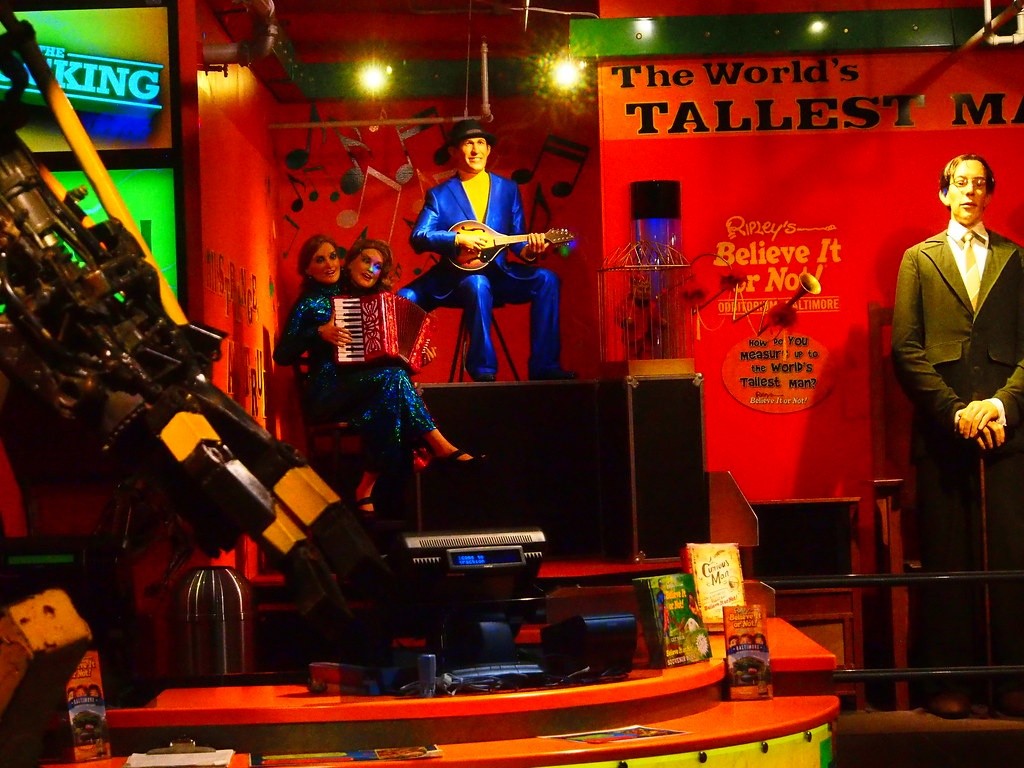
[750,502,858,577]
[416,373,711,564]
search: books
[58,648,111,763]
[681,542,748,635]
[722,605,775,702]
[632,572,714,666]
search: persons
[892,153,1024,720]
[613,271,667,362]
[409,118,580,382]
[272,232,490,527]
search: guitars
[444,219,574,272]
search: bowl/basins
[308,662,364,690]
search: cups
[417,654,436,698]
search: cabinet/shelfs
[775,587,865,710]
